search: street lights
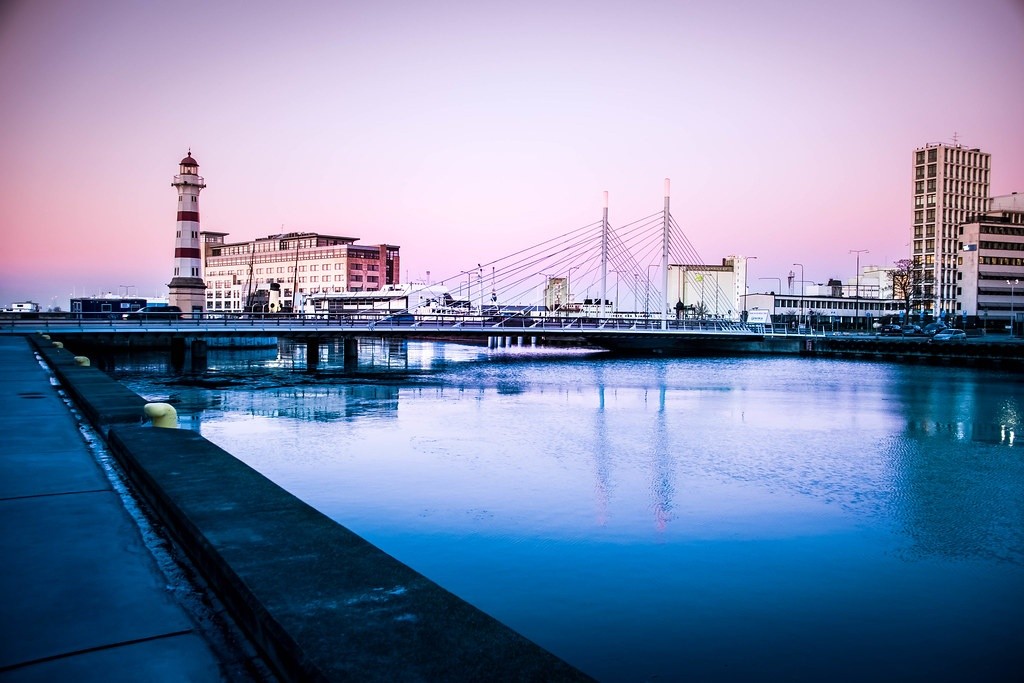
[695,272,712,320]
[850,249,869,330]
[646,265,662,320]
[743,257,757,320]
[1006,279,1019,338]
[634,274,639,318]
[539,272,555,322]
[794,264,804,326]
[608,270,628,318]
[461,270,478,315]
[568,267,580,317]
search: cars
[500,316,534,327]
[380,311,414,321]
[933,329,966,343]
[881,323,947,338]
[122,306,182,322]
